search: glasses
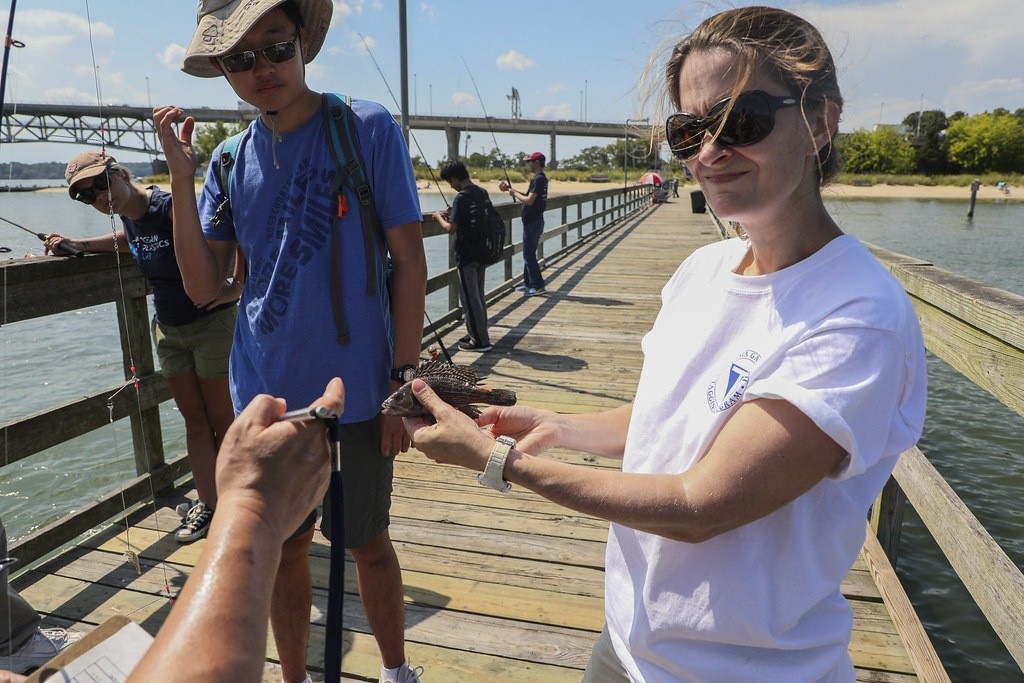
[74,166,112,205]
[666,89,801,160]
[216,26,299,75]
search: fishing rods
[459,53,517,204]
[0,0,27,128]
[424,311,454,365]
[357,30,453,212]
[0,216,86,259]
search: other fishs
[380,361,517,424]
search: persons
[432,161,492,353]
[673,179,679,198]
[499,152,548,296]
[0,377,346,682]
[44,153,246,543]
[151,1,427,683]
[0,519,86,674]
[402,7,927,683]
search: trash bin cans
[691,190,706,213]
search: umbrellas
[638,172,663,201]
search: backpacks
[458,188,507,267]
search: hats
[181,0,333,78]
[523,152,545,163]
[65,152,117,200]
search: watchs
[476,434,516,492]
[391,364,418,385]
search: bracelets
[231,276,244,289]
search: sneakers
[515,282,529,292]
[175,499,214,542]
[0,626,87,674]
[458,334,492,352]
[524,287,546,296]
[379,657,425,683]
